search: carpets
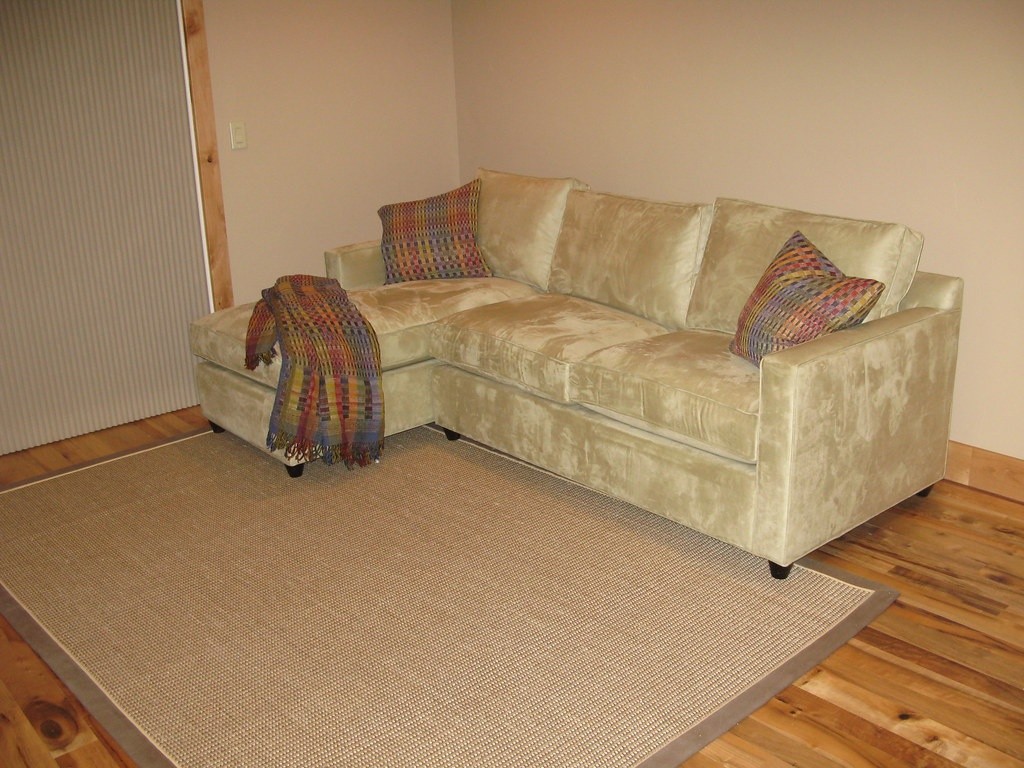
[0,423,902,768]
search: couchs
[187,238,964,579]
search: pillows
[377,177,493,286]
[729,232,886,369]
[685,196,925,336]
[546,188,712,331]
[475,166,592,295]
